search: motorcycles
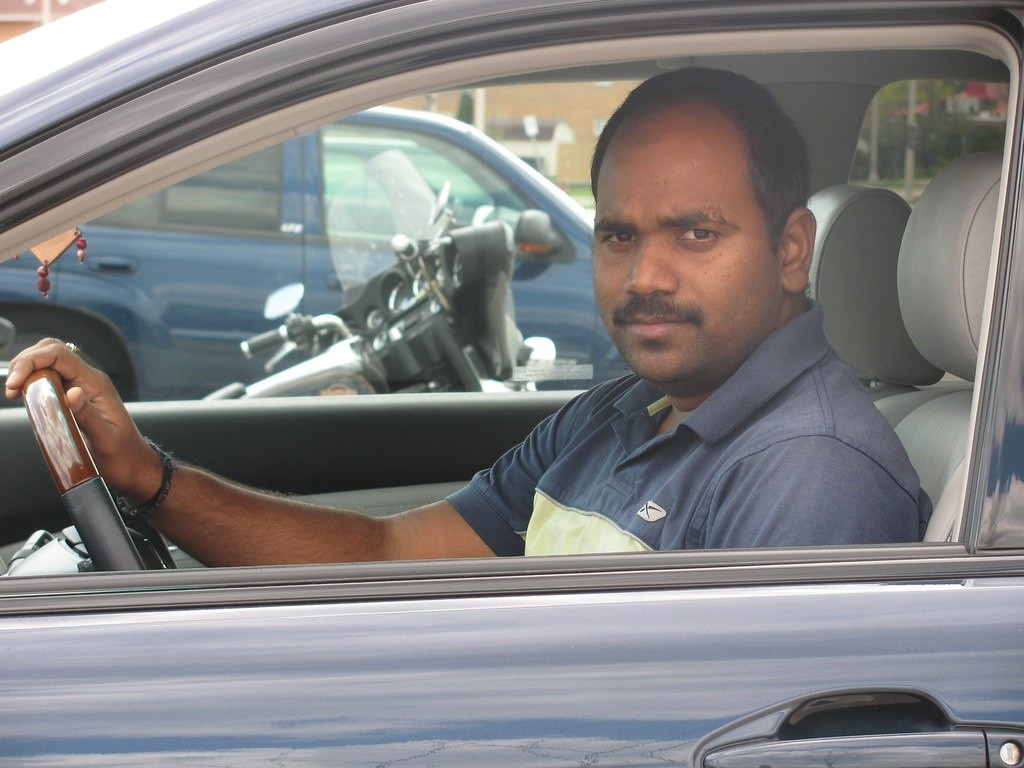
[3,191,563,582]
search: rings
[66,343,79,353]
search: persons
[5,68,929,571]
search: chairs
[802,151,1005,542]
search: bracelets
[115,436,176,517]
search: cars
[0,101,635,409]
[0,0,1024,768]
